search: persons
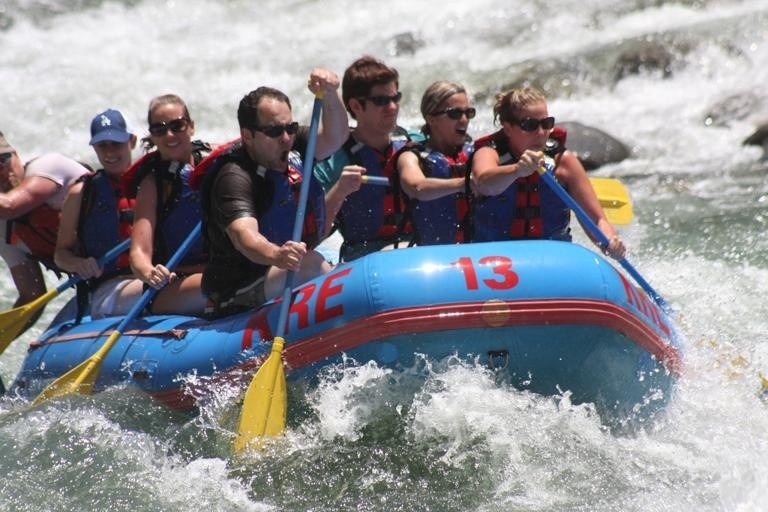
[188,67,350,321]
[466,88,626,260]
[118,95,219,315]
[391,80,475,246]
[53,109,146,321]
[0,131,95,340]
[313,56,428,264]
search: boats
[7,240,682,422]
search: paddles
[235,89,324,454]
[360,174,633,225]
[32,221,204,407]
[0,235,134,355]
[535,166,768,410]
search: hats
[0,132,16,155]
[89,108,132,144]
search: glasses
[255,121,299,139]
[360,92,401,106]
[510,116,554,132]
[149,116,189,138]
[433,107,476,120]
